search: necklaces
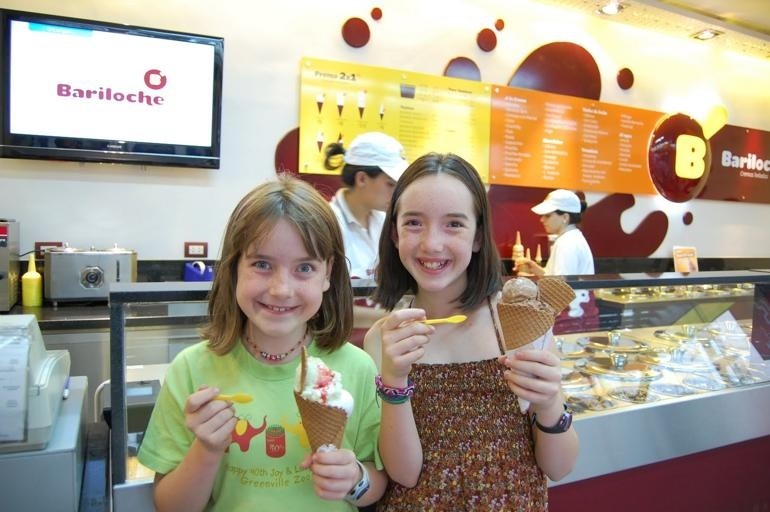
[237,321,309,365]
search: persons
[363,151,580,511]
[135,163,390,512]
[511,189,600,333]
[326,134,409,277]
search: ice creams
[497,276,577,350]
[314,88,387,150]
[293,345,355,453]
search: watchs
[345,457,371,502]
[534,398,573,433]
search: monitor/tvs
[0,8,224,169]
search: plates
[557,321,770,414]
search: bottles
[20,252,44,308]
[512,229,524,260]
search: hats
[530,188,582,216]
[343,131,410,183]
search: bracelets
[375,372,416,399]
[374,388,410,404]
